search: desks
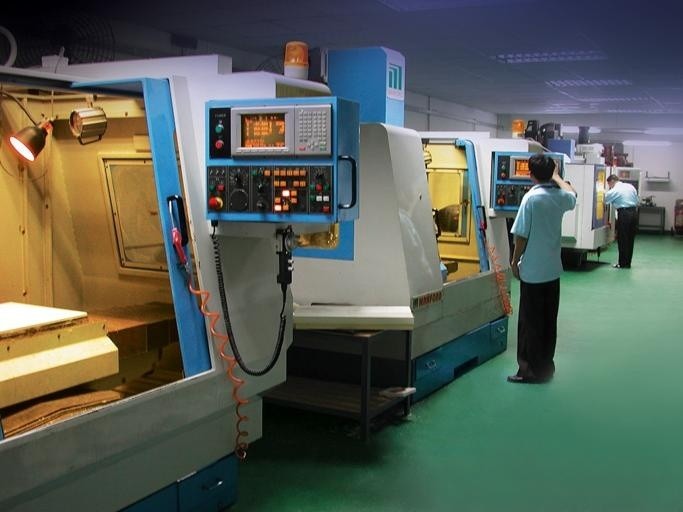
[637,205,666,235]
[262,330,413,440]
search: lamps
[433,204,460,238]
[0,87,54,163]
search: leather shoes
[508,375,544,384]
[616,264,631,268]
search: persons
[506,155,577,383]
[604,175,639,267]
